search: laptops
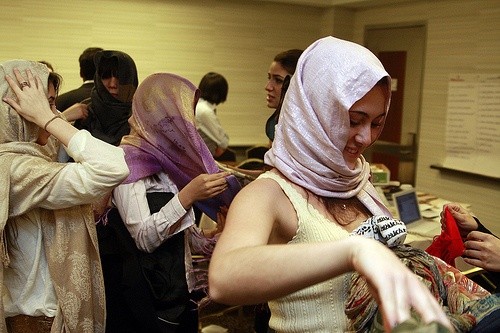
[392,189,443,237]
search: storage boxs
[367,164,390,186]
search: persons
[440,202,500,272]
[0,47,305,333]
[208,36,454,333]
[0,59,129,333]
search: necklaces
[341,202,346,210]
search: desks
[381,187,482,275]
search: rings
[21,82,28,88]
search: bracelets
[45,116,63,129]
